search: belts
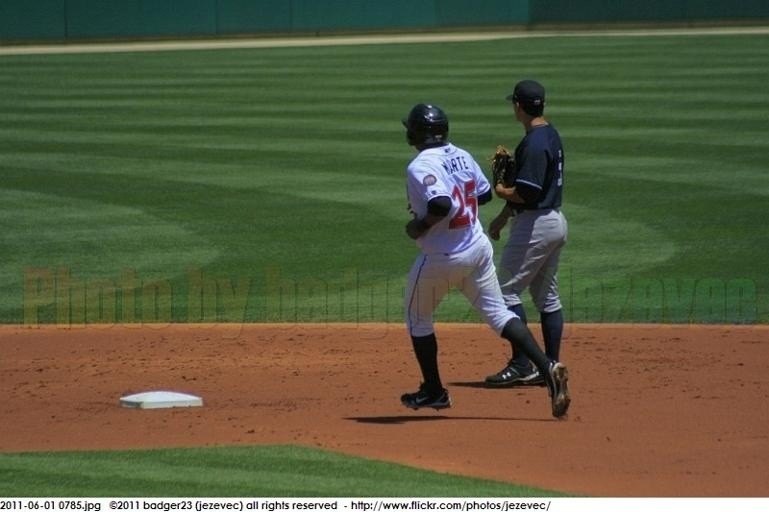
[510,207,527,217]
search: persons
[479,78,571,389]
[398,101,574,418]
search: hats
[506,80,545,106]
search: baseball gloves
[492,145,515,188]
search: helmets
[403,103,448,145]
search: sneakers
[401,383,452,409]
[544,360,571,417]
[486,360,558,384]
